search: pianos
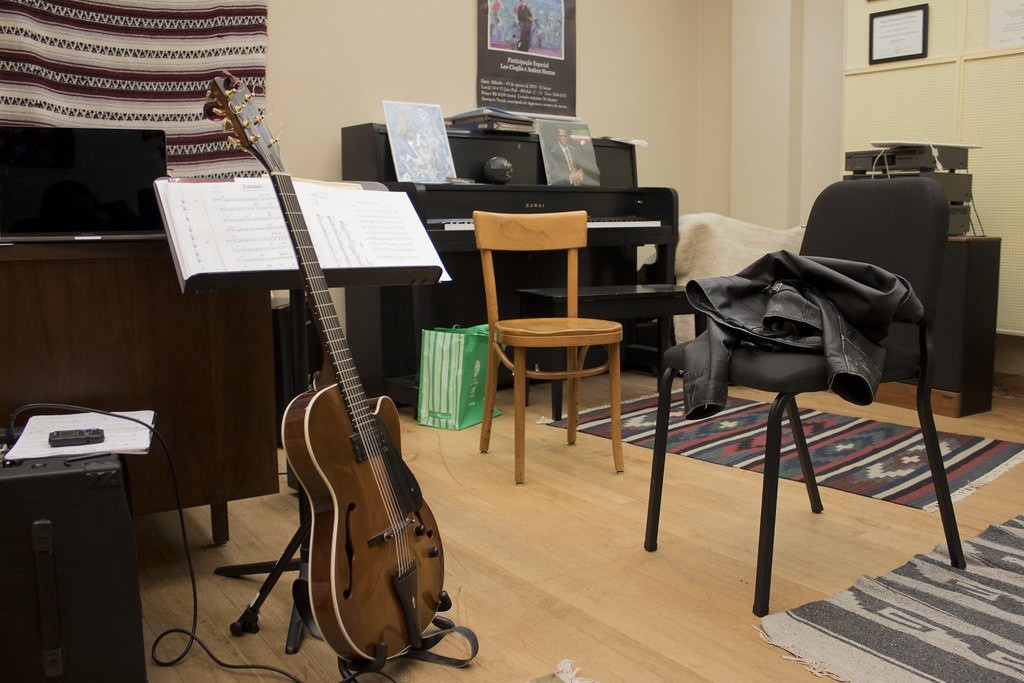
[341,123,680,420]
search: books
[154,177,451,283]
[4,408,157,455]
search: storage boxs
[414,321,502,434]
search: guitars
[202,75,446,662]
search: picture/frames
[867,2,929,65]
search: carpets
[534,380,1023,516]
[749,507,1022,680]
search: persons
[550,127,586,186]
[516,0,532,53]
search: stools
[513,282,712,422]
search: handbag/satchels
[417,321,502,430]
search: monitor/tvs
[0,125,166,237]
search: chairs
[470,209,627,487]
[641,173,967,619]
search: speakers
[0,427,149,683]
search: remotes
[49,429,104,446]
